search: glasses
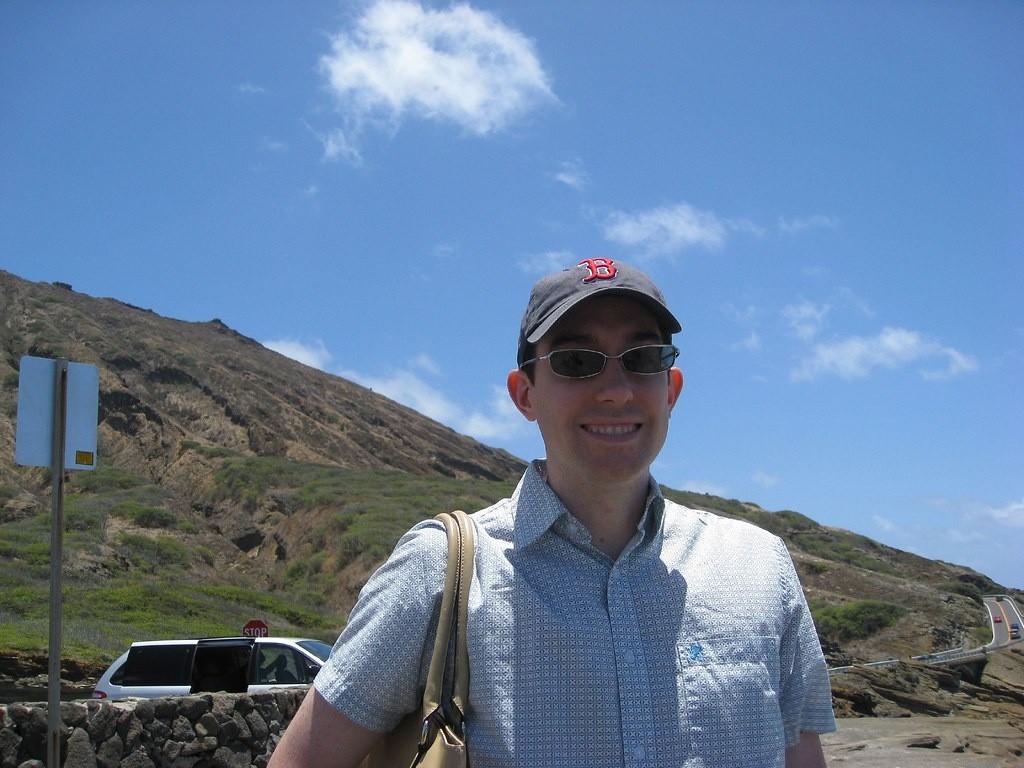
[518,344,680,379]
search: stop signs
[242,619,269,638]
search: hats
[517,257,683,363]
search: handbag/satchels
[358,510,474,768]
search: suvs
[89,634,335,702]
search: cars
[1010,623,1020,639]
[993,616,1002,623]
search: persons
[258,651,282,679]
[267,257,837,768]
